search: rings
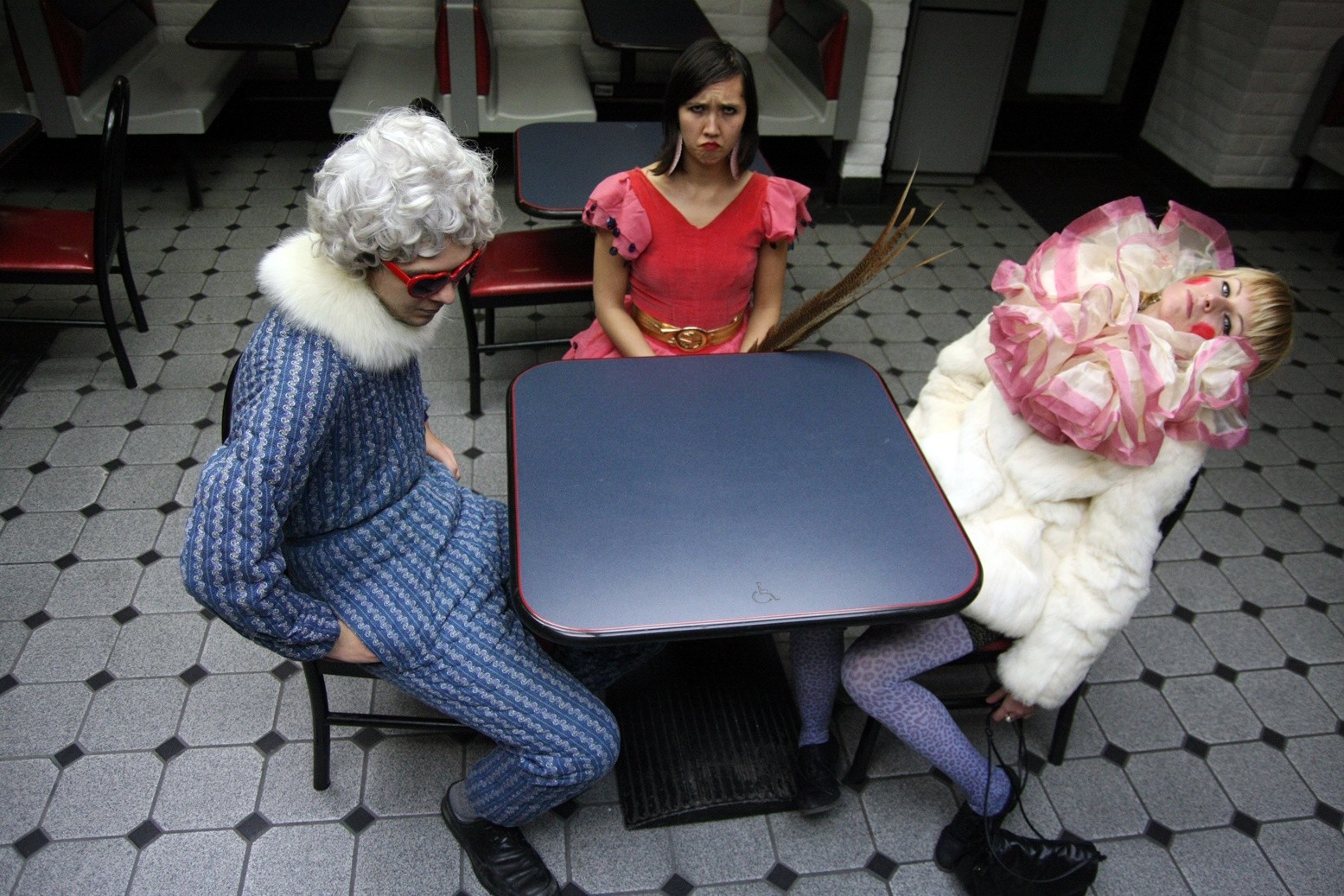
[1006,715,1011,721]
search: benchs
[0,0,854,179]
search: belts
[629,305,744,351]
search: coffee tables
[505,347,986,835]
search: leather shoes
[440,777,563,896]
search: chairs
[407,97,638,420]
[0,75,149,390]
[845,301,1199,792]
[223,339,580,823]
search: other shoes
[932,764,1023,873]
[797,730,842,816]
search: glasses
[380,248,484,299]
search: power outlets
[596,84,613,96]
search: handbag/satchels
[953,823,1104,896]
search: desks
[579,0,723,110]
[185,0,352,124]
[0,112,42,160]
[511,119,780,221]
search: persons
[788,196,1294,872]
[563,39,812,360]
[178,105,618,895]
